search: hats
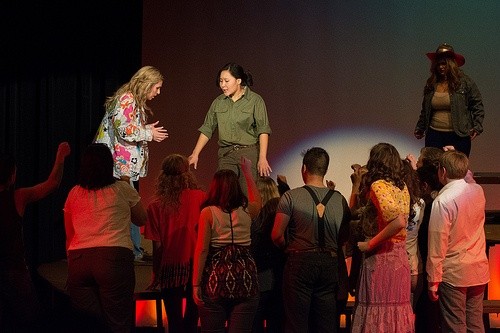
[424,44,466,67]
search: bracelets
[193,284,201,288]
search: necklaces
[414,44,483,160]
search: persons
[96,66,168,263]
[0,141,488,333]
[189,62,274,196]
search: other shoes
[136,252,152,262]
